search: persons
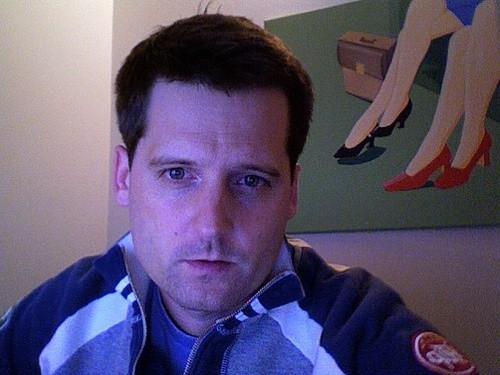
[1,0,481,375]
